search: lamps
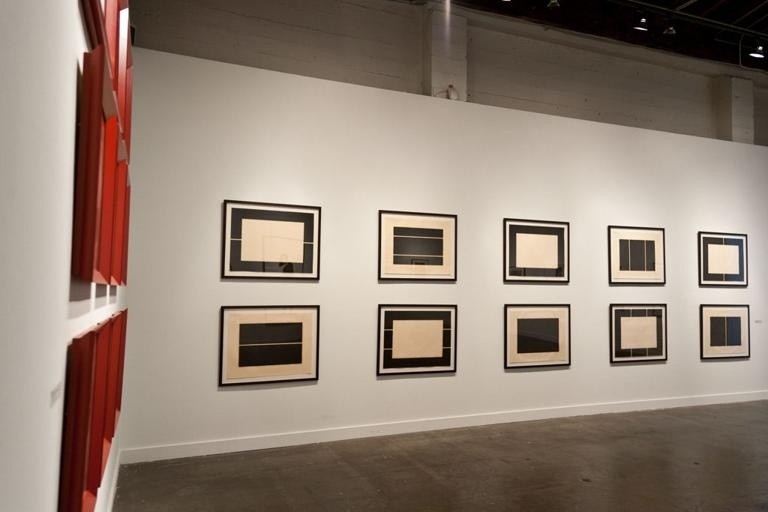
[747,37,766,60]
[631,11,651,32]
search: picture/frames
[375,208,459,377]
[216,199,323,388]
[501,217,573,371]
[697,230,752,361]
[606,223,670,364]
